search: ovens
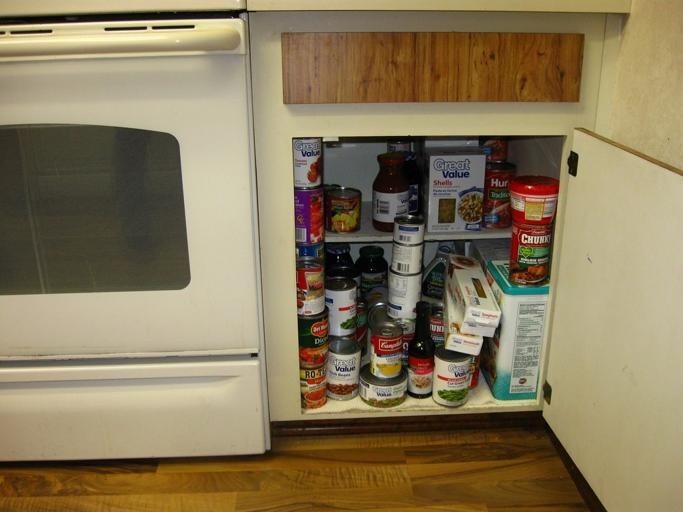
[0,20,264,364]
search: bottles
[407,300,436,399]
[324,241,389,297]
[406,153,423,217]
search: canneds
[292,136,553,409]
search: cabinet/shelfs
[244,16,683,512]
